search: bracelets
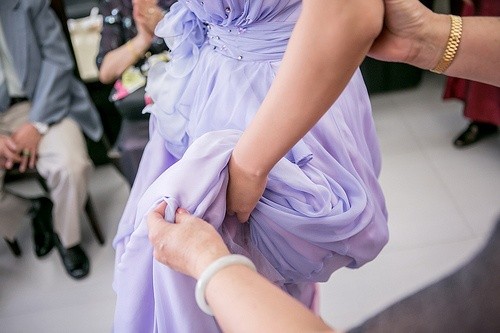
[430,15,463,74]
[128,41,140,61]
[196,254,257,317]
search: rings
[20,149,29,156]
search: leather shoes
[57,241,89,278]
[452,121,496,146]
[29,196,55,255]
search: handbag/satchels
[111,58,151,119]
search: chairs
[3,0,104,257]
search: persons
[442,0,500,147]
[147,0,500,333]
[0,0,104,279]
[97,1,177,190]
[112,0,390,333]
